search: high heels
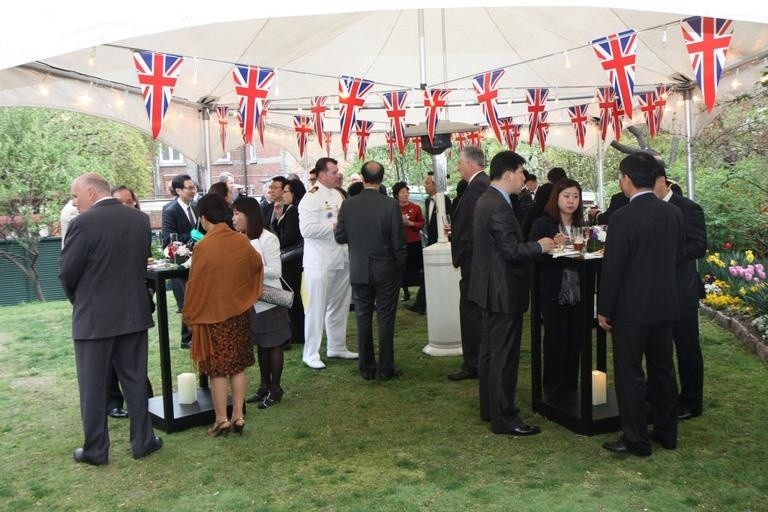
[230,417,245,436]
[207,420,231,438]
[247,383,269,403]
[258,386,284,409]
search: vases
[170,253,187,266]
[586,238,604,253]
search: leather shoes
[74,448,107,465]
[107,407,128,418]
[326,349,359,359]
[404,305,426,315]
[491,421,540,435]
[647,428,677,449]
[602,437,652,457]
[361,366,377,379]
[678,407,690,419]
[378,368,401,378]
[401,295,410,301]
[448,370,474,381]
[133,436,163,460]
[304,355,327,369]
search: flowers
[168,240,193,258]
[589,225,607,243]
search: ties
[187,206,195,227]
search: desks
[144,259,246,435]
[529,240,654,434]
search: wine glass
[441,215,452,236]
[169,232,179,268]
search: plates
[146,262,178,271]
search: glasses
[184,186,197,192]
[119,200,137,206]
[308,178,316,182]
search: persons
[58,145,583,417]
[56,171,165,465]
[466,150,560,435]
[180,190,264,438]
[596,150,684,459]
[646,156,709,421]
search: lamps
[592,369,608,406]
[177,372,198,405]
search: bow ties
[428,196,436,202]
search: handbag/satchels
[281,245,304,262]
[258,283,294,309]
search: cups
[572,226,583,251]
